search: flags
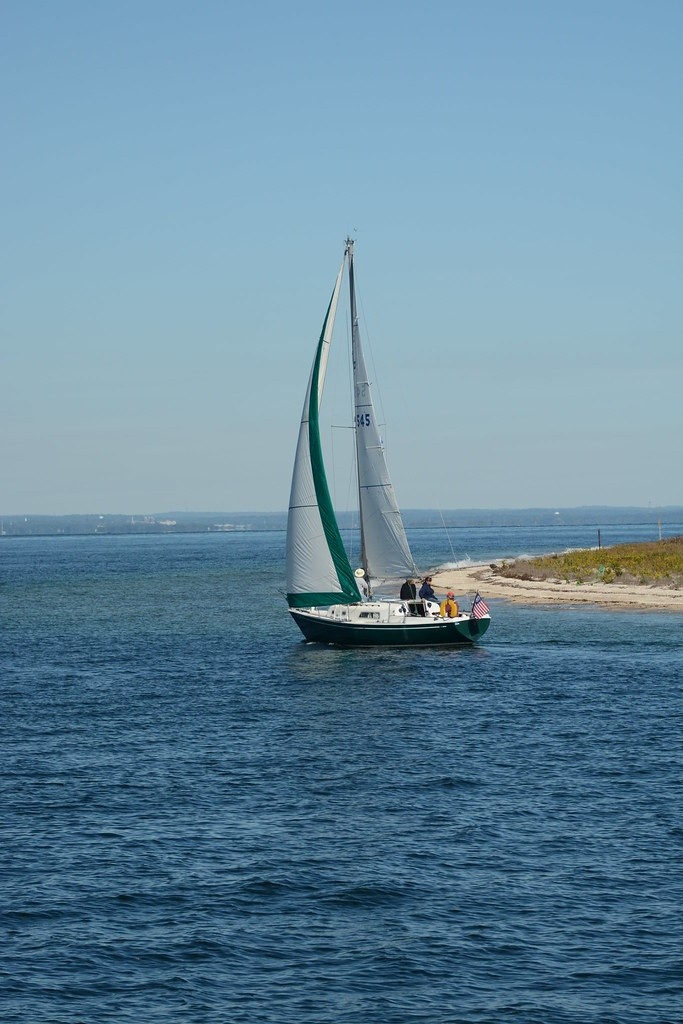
[472,593,490,619]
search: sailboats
[284,236,493,648]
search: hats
[447,593,455,597]
[355,568,365,578]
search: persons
[354,566,371,603]
[400,578,417,616]
[445,592,459,618]
[419,577,440,616]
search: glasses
[427,580,431,582]
